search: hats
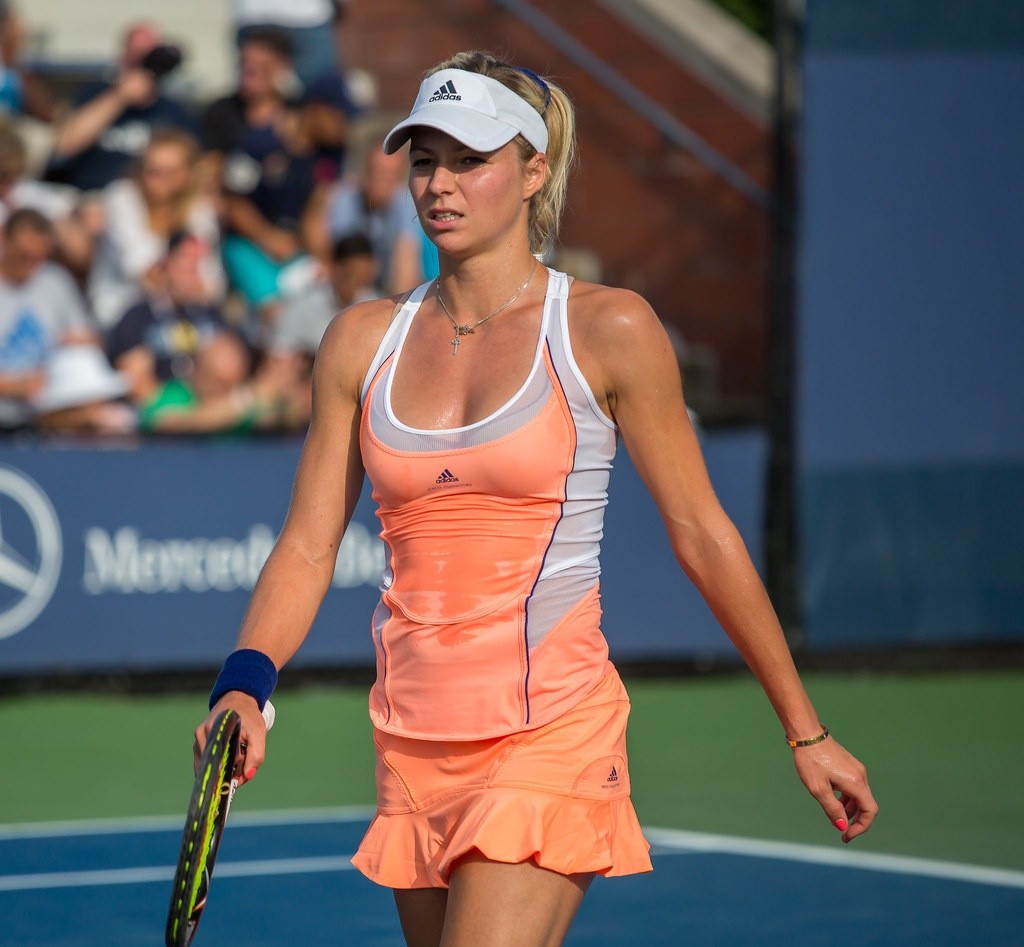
[284,67,361,119]
[32,344,131,412]
[383,67,548,155]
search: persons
[0,0,446,446]
[192,49,879,947]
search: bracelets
[784,724,830,747]
[209,646,278,711]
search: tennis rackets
[163,698,278,947]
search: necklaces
[433,273,536,356]
